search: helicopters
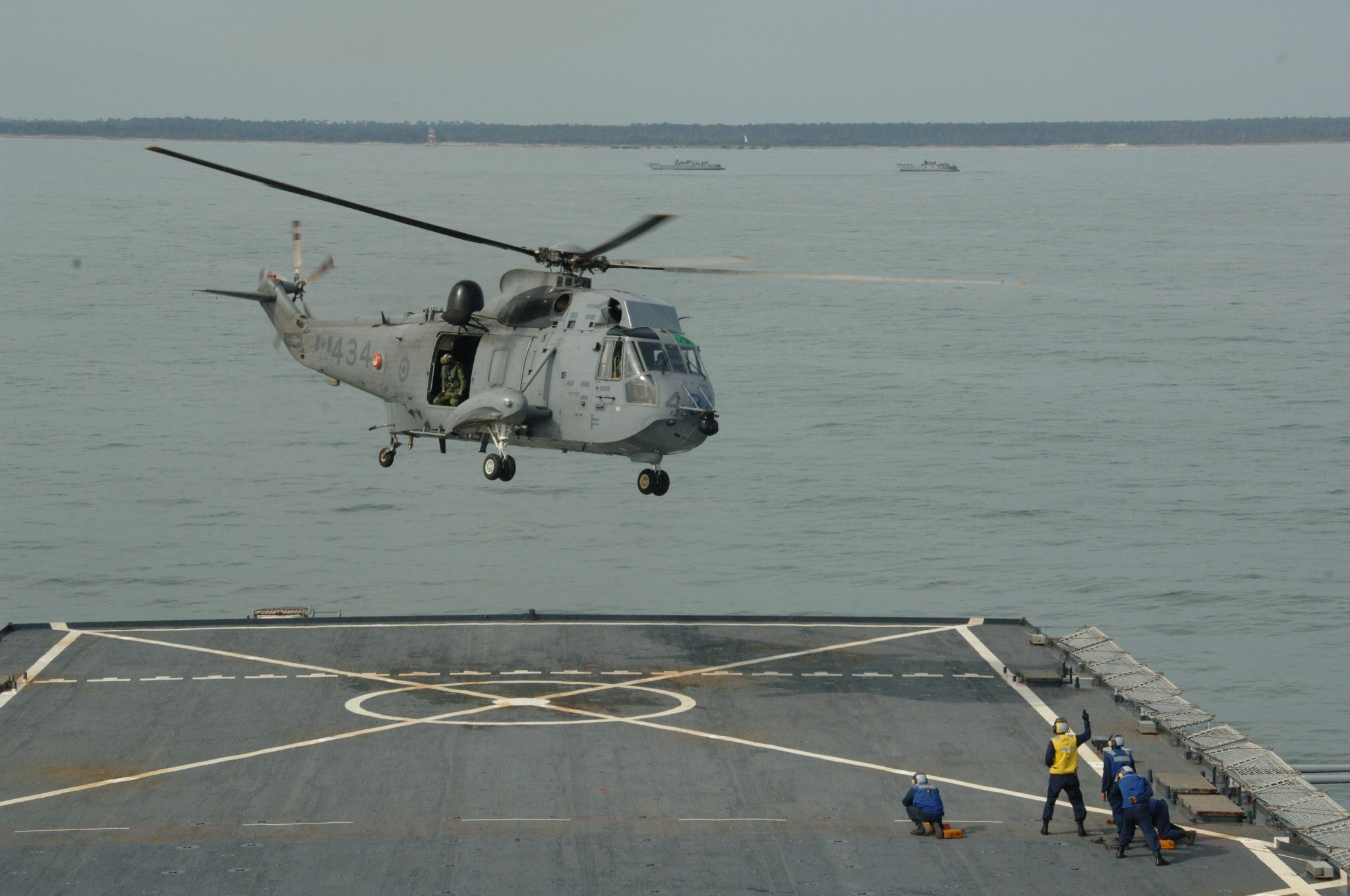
[142,144,1024,495]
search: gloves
[1082,709,1089,721]
[437,392,445,398]
[453,393,461,399]
[1128,796,1137,805]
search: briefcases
[941,829,963,839]
[1155,834,1175,849]
[924,822,951,833]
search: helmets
[1053,718,1070,734]
[911,771,928,785]
[1118,766,1136,779]
[1108,733,1125,748]
[440,354,455,367]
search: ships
[895,157,960,172]
[648,156,726,170]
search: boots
[933,821,944,839]
[1111,842,1130,850]
[1116,845,1124,858]
[1040,819,1050,835]
[1185,830,1196,846]
[1154,851,1168,866]
[1077,820,1087,837]
[910,820,926,836]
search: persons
[433,353,466,406]
[1108,766,1171,866]
[1099,732,1137,841]
[1105,799,1196,846]
[902,772,946,839]
[1040,708,1091,836]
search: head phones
[1108,733,1124,746]
[912,771,928,785]
[1053,718,1071,735]
[1118,766,1136,778]
